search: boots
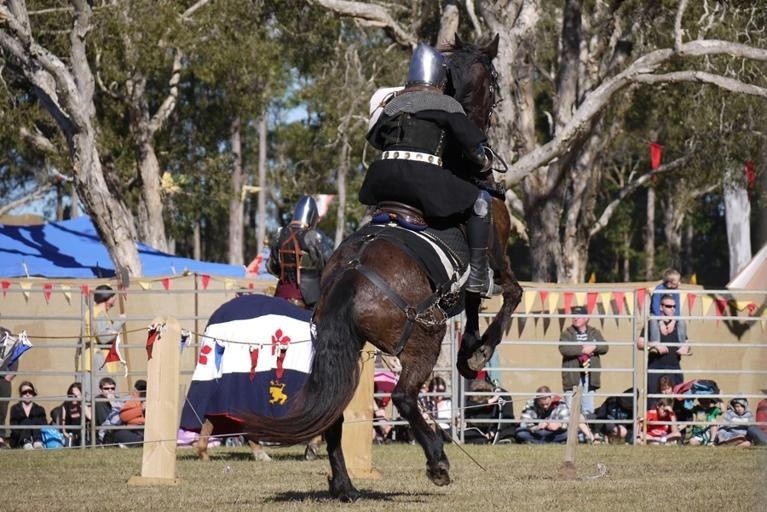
[465,216,504,294]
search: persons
[265,195,334,313]
[560,306,608,414]
[1,284,147,447]
[468,378,636,444]
[357,44,504,298]
[375,353,452,443]
[636,269,691,407]
[648,374,766,447]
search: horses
[238,28,525,501]
[198,294,326,465]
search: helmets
[292,195,319,229]
[407,40,449,91]
[94,285,114,303]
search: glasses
[22,390,33,394]
[103,388,116,390]
[665,305,676,308]
